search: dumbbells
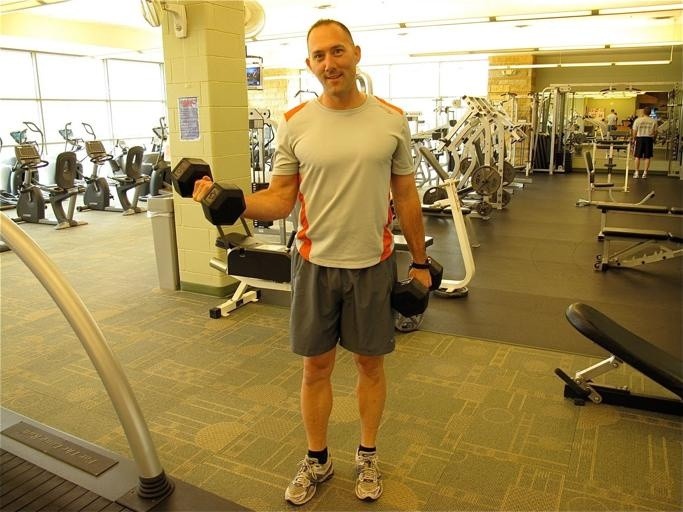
[171,158,246,225]
[389,256,443,318]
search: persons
[192,20,433,505]
[632,107,658,179]
[606,109,618,131]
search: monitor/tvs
[247,64,264,90]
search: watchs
[412,258,432,269]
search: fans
[141,1,189,38]
[245,1,266,40]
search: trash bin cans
[147,193,179,291]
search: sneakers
[285,450,333,506]
[633,174,647,178]
[355,448,383,501]
